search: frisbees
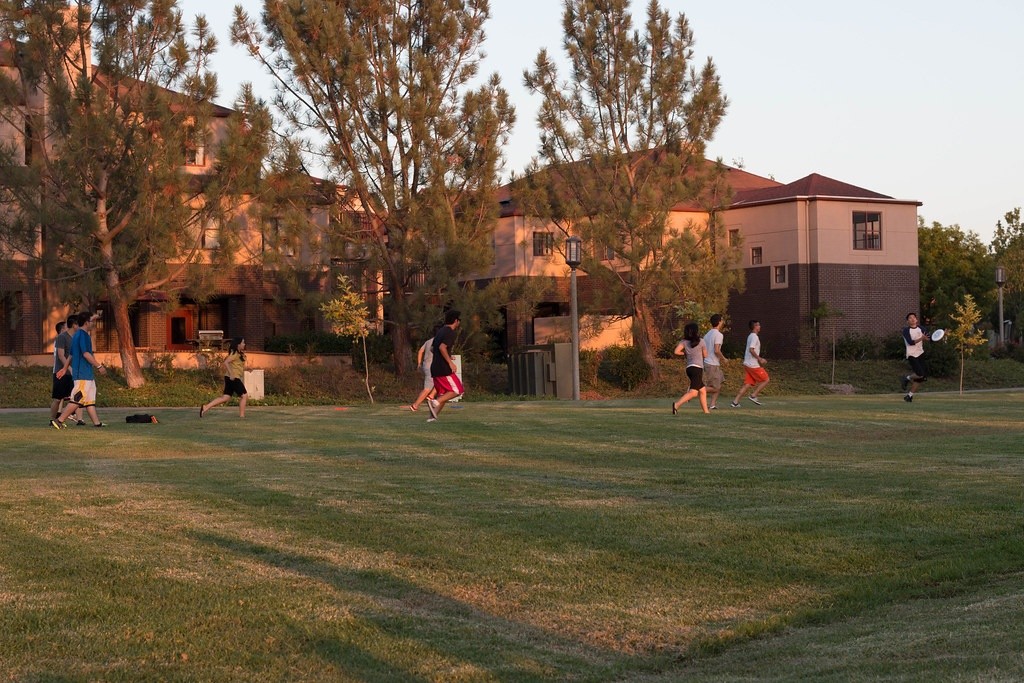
[931,328,944,341]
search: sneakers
[686,386,692,404]
[52,417,68,429]
[901,372,910,391]
[730,401,743,408]
[747,393,762,405]
[904,394,914,402]
[94,422,106,428]
[707,405,718,410]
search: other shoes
[49,419,54,425]
[238,415,247,420]
[76,419,86,425]
[410,404,418,411]
[427,413,437,422]
[200,404,206,417]
[428,400,441,420]
[424,394,434,404]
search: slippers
[672,402,680,415]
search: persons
[410,325,455,411]
[427,311,465,423]
[672,323,711,415]
[731,320,770,408]
[901,313,948,402]
[686,314,729,409]
[49,312,108,429]
[200,336,248,417]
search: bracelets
[97,365,102,369]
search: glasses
[755,324,760,326]
[457,318,460,322]
[90,319,94,322]
